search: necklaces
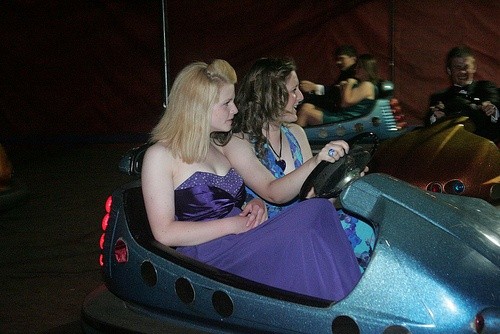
[258,127,286,172]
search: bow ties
[453,84,472,94]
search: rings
[328,148,335,157]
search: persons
[293,45,362,112]
[140,58,362,301]
[212,57,376,271]
[426,44,500,143]
[296,54,378,128]
